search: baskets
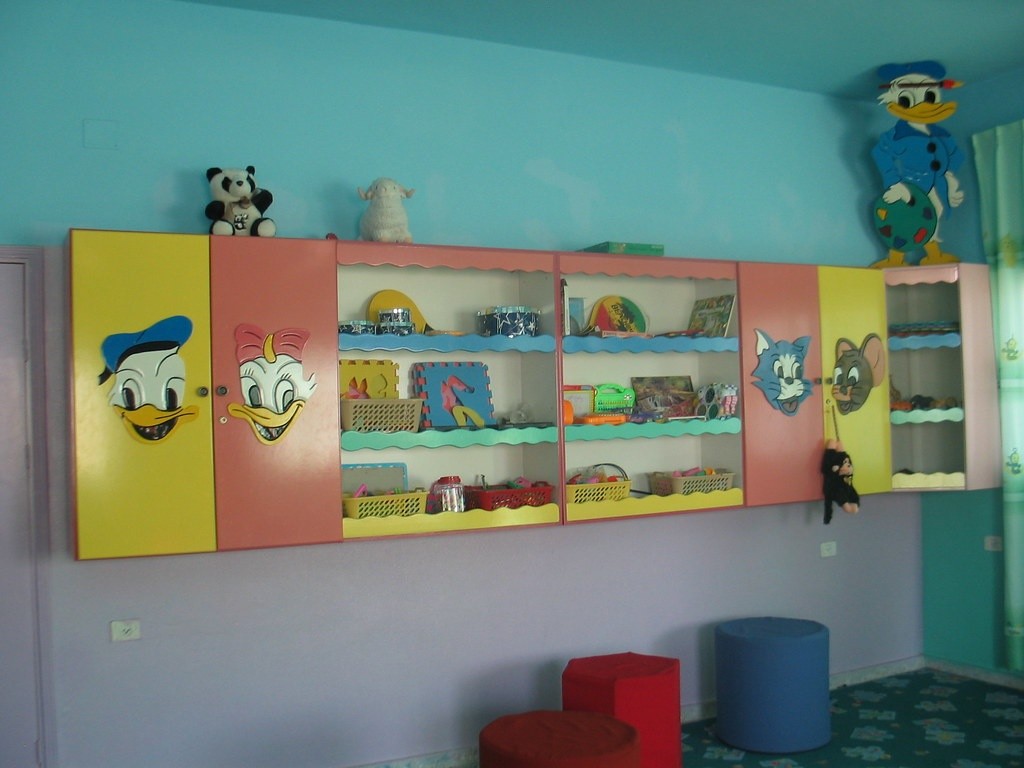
[647,467,734,496]
[340,398,426,435]
[565,475,632,501]
[465,480,555,513]
[342,488,430,523]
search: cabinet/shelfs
[65,227,1002,562]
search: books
[687,295,735,337]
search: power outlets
[111,619,140,641]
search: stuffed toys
[357,177,415,244]
[821,440,860,524]
[206,166,276,237]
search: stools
[715,616,832,753]
[561,652,681,768]
[479,710,638,768]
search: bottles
[435,476,465,515]
